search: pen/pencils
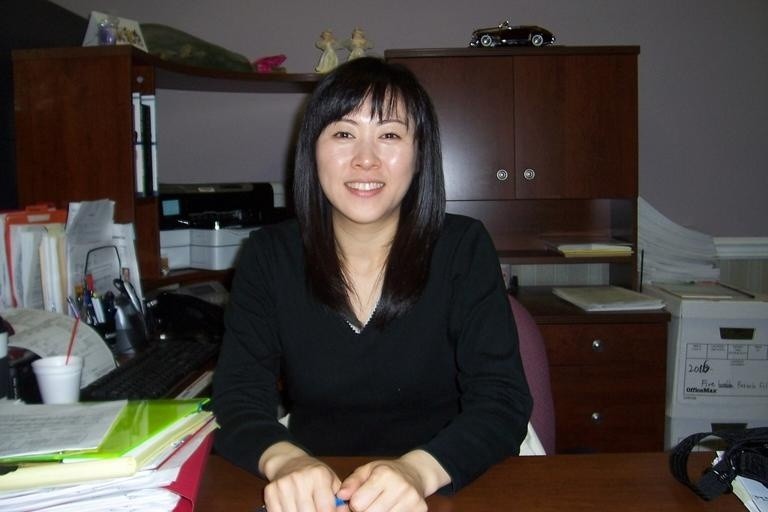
[66,272,114,326]
[261,498,349,508]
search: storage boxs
[642,279,768,422]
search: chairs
[506,296,556,455]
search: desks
[193,436,767,512]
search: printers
[158,180,289,272]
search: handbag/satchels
[727,426,768,486]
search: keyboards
[77,330,217,400]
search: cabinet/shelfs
[0,43,163,282]
[509,284,673,455]
[385,40,641,290]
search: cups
[32,355,84,402]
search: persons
[207,55,533,512]
